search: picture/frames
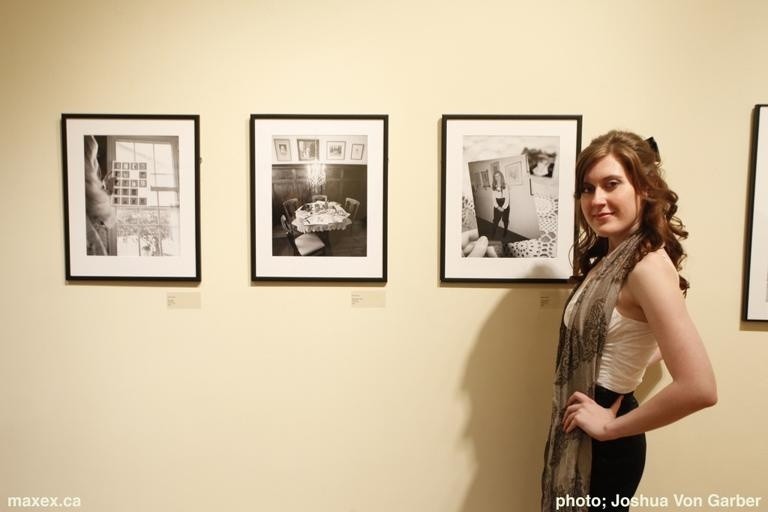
[250,114,389,283]
[61,113,201,281]
[742,104,768,322]
[440,115,582,284]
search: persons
[492,171,510,237]
[541,130,718,512]
[461,228,498,258]
